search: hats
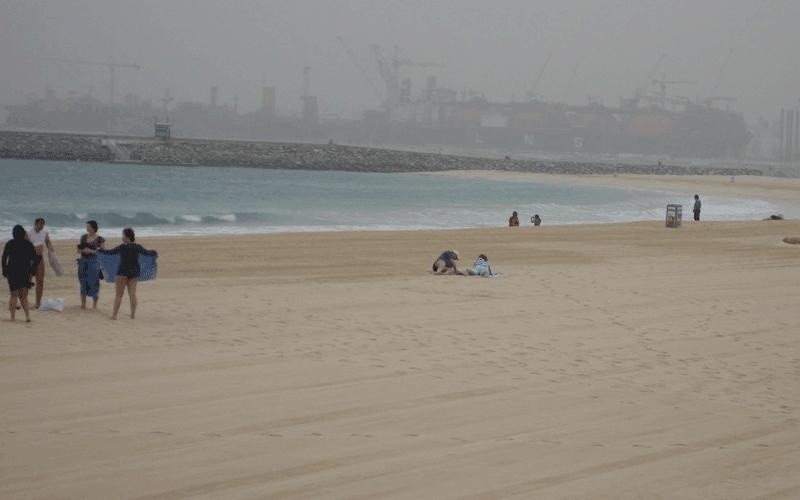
[451,250,460,260]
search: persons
[75,220,106,311]
[1,223,41,324]
[465,253,499,277]
[95,227,159,321]
[530,214,541,226]
[508,211,519,227]
[13,218,55,312]
[433,249,462,275]
[692,194,702,221]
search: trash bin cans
[666,204,683,228]
